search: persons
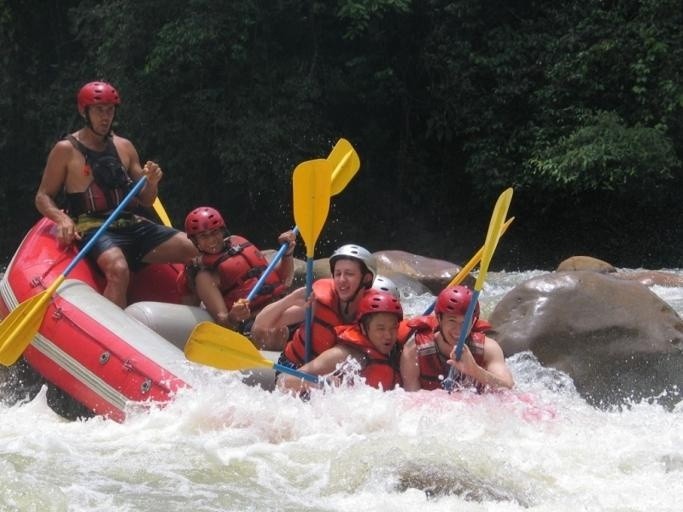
[277,292,403,390]
[186,206,318,351]
[251,244,377,402]
[374,275,400,298]
[36,82,199,308]
[399,286,514,392]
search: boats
[0,209,281,424]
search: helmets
[370,275,400,299]
[185,207,226,238]
[329,244,376,289]
[356,291,403,324]
[435,286,480,320]
[78,82,121,113]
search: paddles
[440,187,516,394]
[183,320,329,389]
[0,174,149,367]
[400,216,517,349]
[245,136,362,303]
[291,158,333,365]
